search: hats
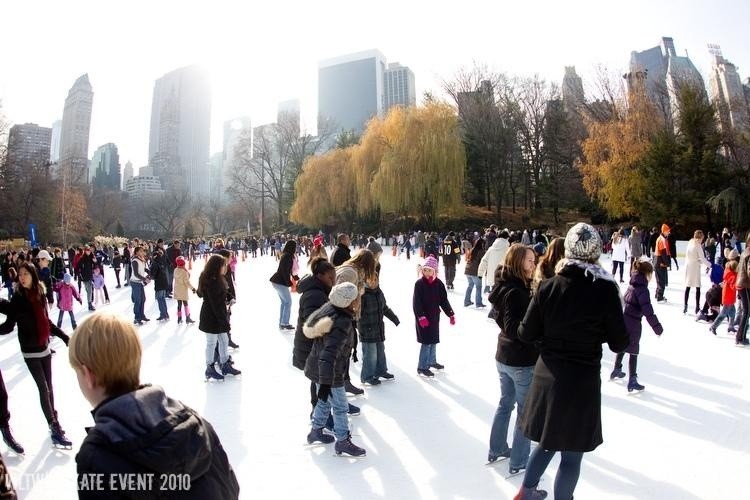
[564,222,603,260]
[366,236,383,254]
[64,273,70,282]
[661,223,670,234]
[176,256,185,266]
[328,281,359,308]
[421,254,438,277]
[36,249,53,261]
[728,247,737,260]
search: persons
[0,221,750,500]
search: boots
[610,364,626,379]
[628,377,644,392]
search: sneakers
[306,362,444,458]
[279,324,295,330]
[49,427,71,446]
[683,307,750,346]
[488,448,548,500]
[655,293,667,303]
[447,283,492,307]
[134,291,195,325]
[205,333,241,380]
[4,437,24,453]
[57,283,130,331]
